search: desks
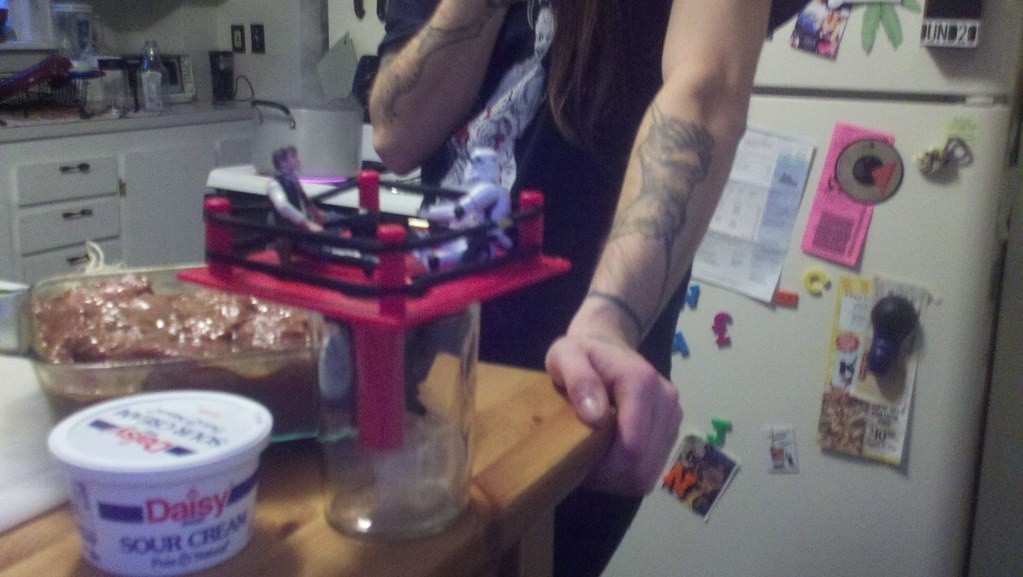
[0,350,622,576]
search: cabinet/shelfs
[0,122,264,286]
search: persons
[370,0,806,577]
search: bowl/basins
[16,264,355,437]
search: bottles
[136,39,170,118]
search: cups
[314,306,482,544]
[0,280,32,355]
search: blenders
[50,0,101,90]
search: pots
[247,93,364,186]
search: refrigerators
[603,0,1023,577]
